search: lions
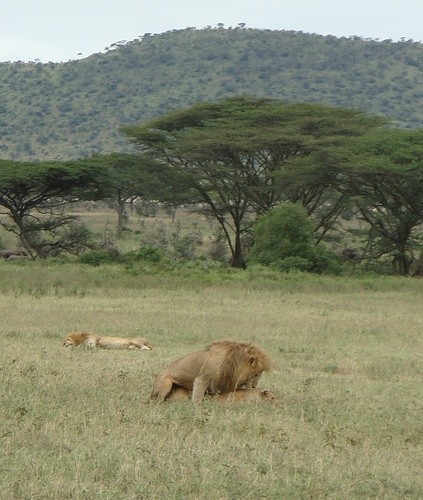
[62,331,153,352]
[149,337,276,410]
